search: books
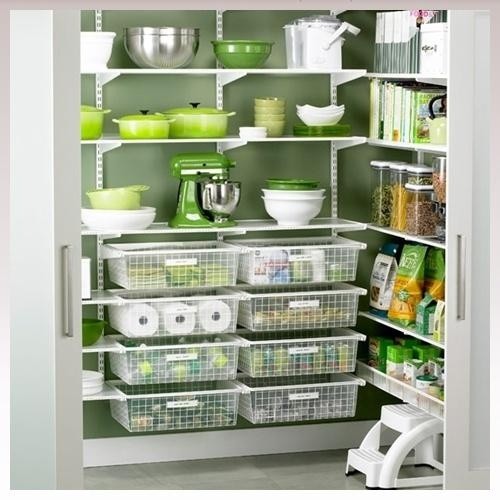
[370,12,446,143]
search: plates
[290,125,352,138]
[82,369,104,396]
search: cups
[425,115,446,145]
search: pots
[80,99,236,141]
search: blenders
[165,151,242,229]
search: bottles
[365,151,446,244]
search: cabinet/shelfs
[79,9,373,440]
[352,11,446,425]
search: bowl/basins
[80,183,158,233]
[209,39,275,66]
[80,30,116,69]
[252,95,288,138]
[259,179,328,229]
[121,26,201,69]
[82,317,108,346]
[293,102,346,126]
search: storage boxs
[103,377,248,435]
[106,333,250,386]
[106,287,239,338]
[107,241,242,291]
[234,282,368,333]
[225,233,368,284]
[234,371,367,425]
[235,327,366,379]
